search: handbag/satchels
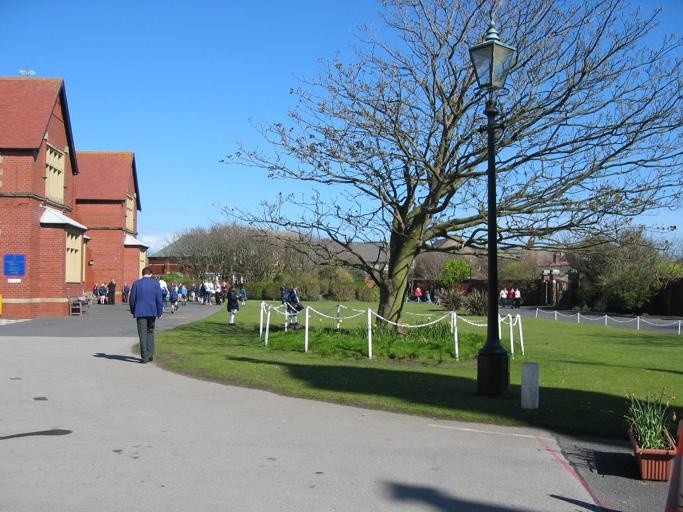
[296,303,305,312]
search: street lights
[467,11,518,343]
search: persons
[279,284,300,325]
[415,285,522,310]
[129,267,163,364]
[92,275,247,327]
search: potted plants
[621,383,680,482]
